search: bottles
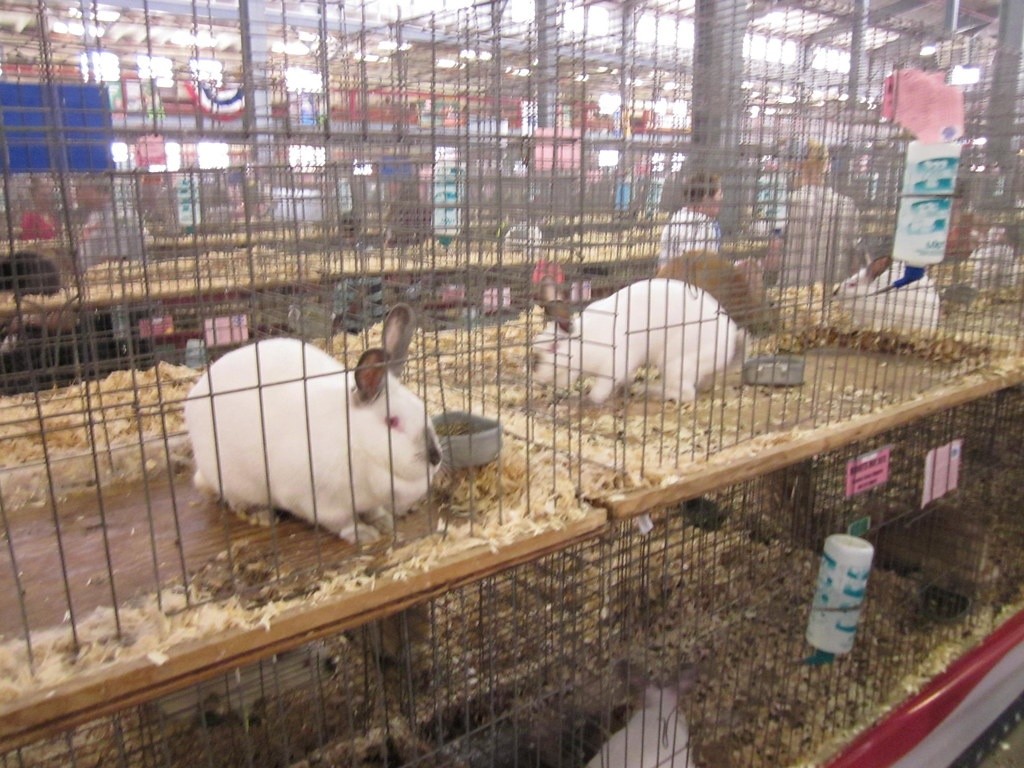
[805,533,875,660]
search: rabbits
[583,654,701,768]
[830,252,940,338]
[185,303,472,545]
[528,274,750,406]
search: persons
[22,176,55,241]
[943,182,980,263]
[82,174,155,256]
[765,140,860,284]
[660,175,721,269]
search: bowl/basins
[744,354,806,386]
[432,409,502,471]
